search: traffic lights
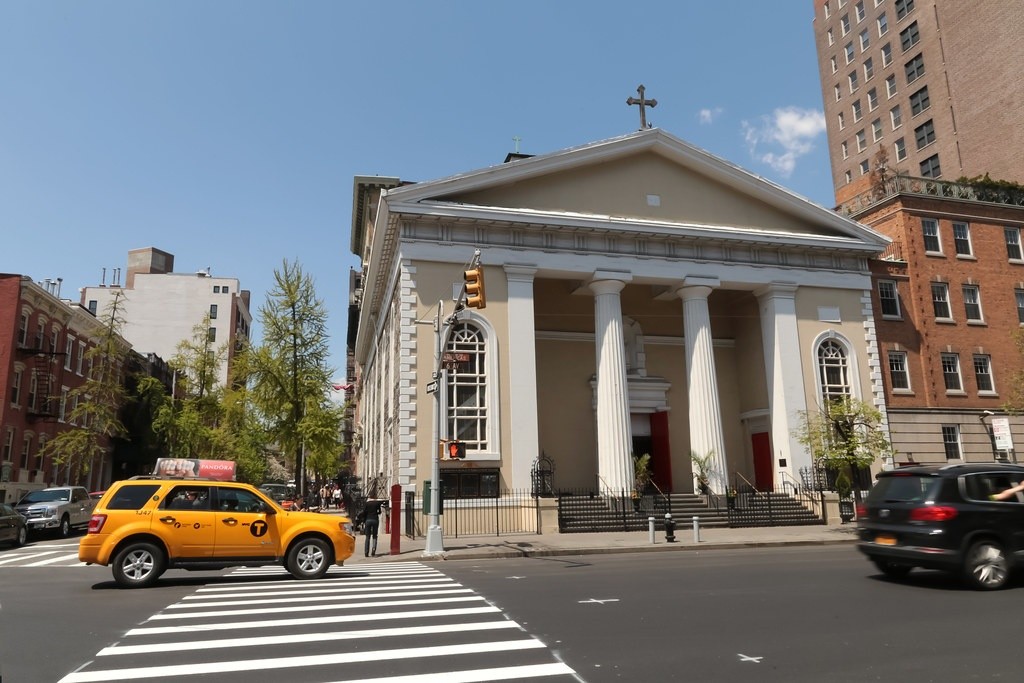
[447,442,466,458]
[463,267,486,309]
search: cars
[0,485,107,547]
[258,484,288,504]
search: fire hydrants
[665,513,676,543]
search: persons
[306,480,343,513]
[225,492,258,512]
[184,490,199,501]
[156,458,200,477]
[988,481,1024,501]
[361,491,382,557]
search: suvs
[79,476,357,588]
[855,461,1024,591]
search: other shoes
[371,551,375,556]
[365,553,368,557]
